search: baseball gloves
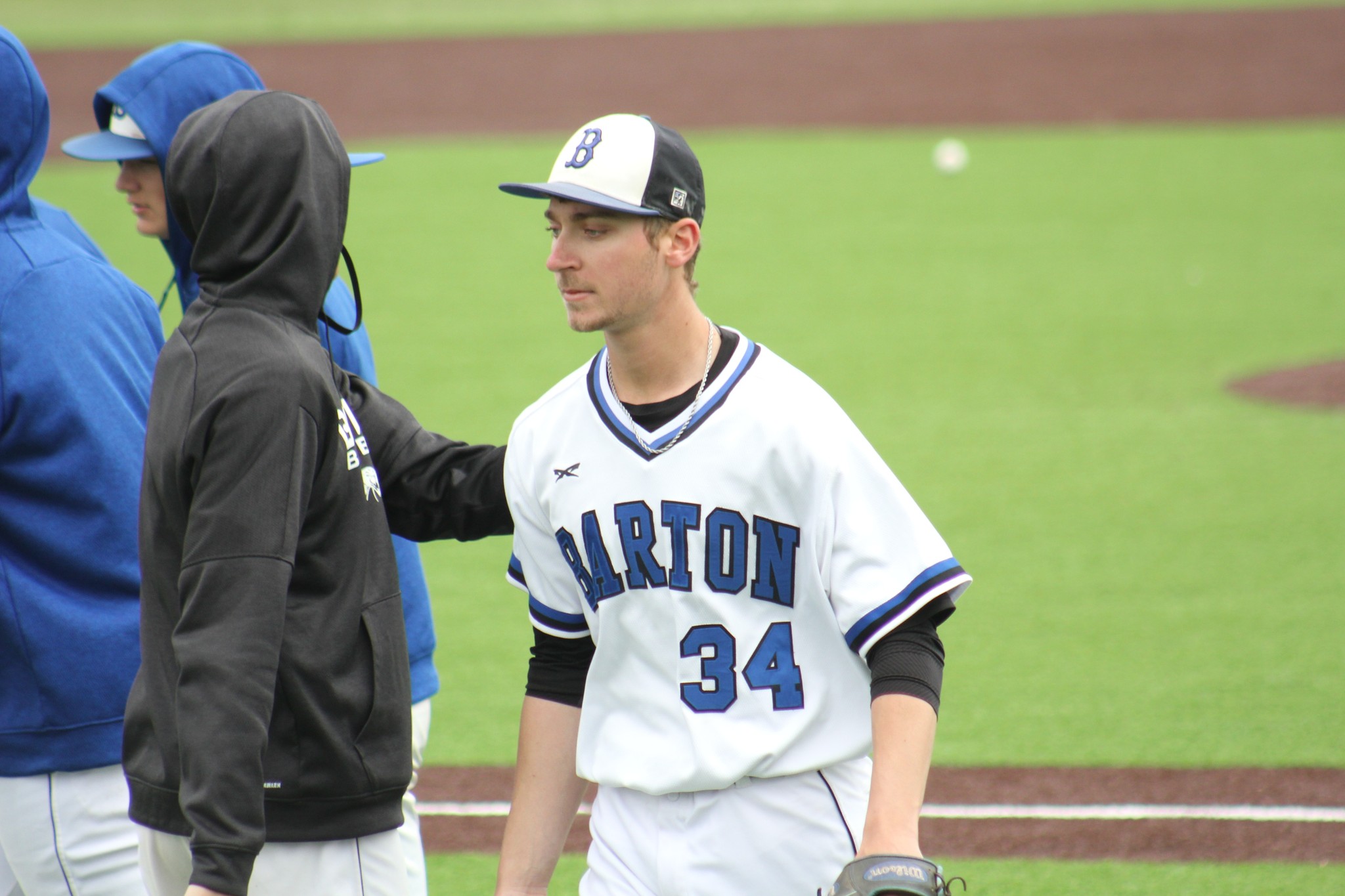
[827,852,951,896]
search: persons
[118,87,514,896]
[497,113,973,896]
[0,29,165,896]
[61,42,441,826]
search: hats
[60,102,156,160]
[498,113,705,230]
[347,152,385,168]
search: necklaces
[606,317,714,453]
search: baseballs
[930,139,967,172]
